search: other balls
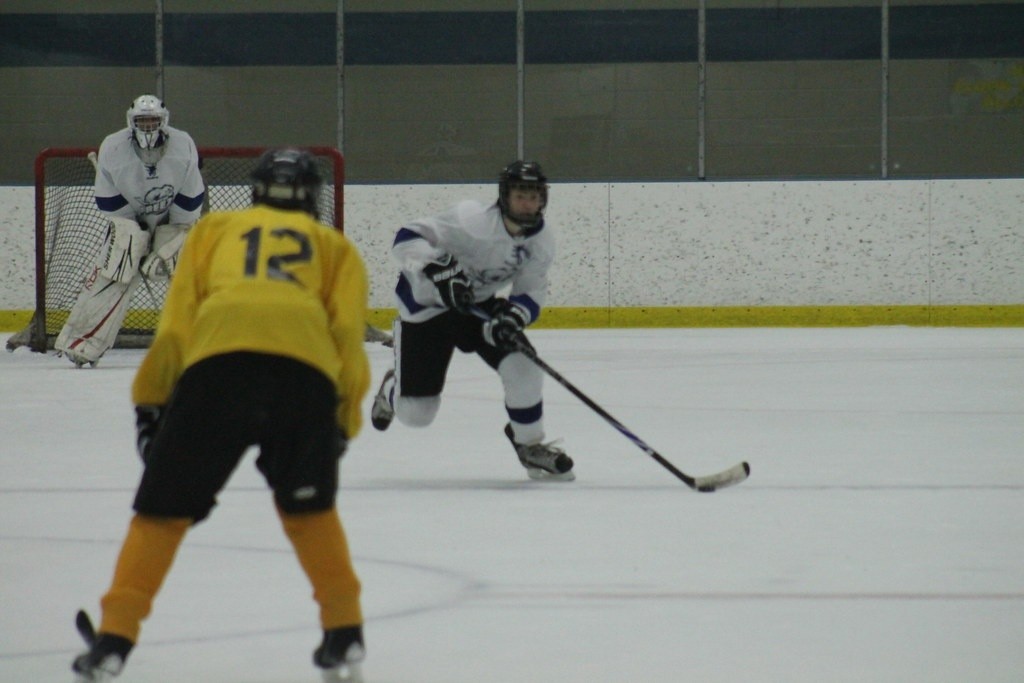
[698,485,716,493]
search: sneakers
[57,350,98,369]
[62,609,132,682]
[313,624,365,682]
[371,370,397,431]
[504,421,575,482]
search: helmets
[126,94,170,151]
[249,145,323,222]
[498,159,548,228]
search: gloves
[481,292,541,350]
[333,427,350,459]
[422,250,476,317]
[135,403,164,464]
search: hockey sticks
[506,337,752,489]
[89,149,99,170]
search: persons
[69,145,374,683]
[51,94,209,369]
[371,157,578,486]
